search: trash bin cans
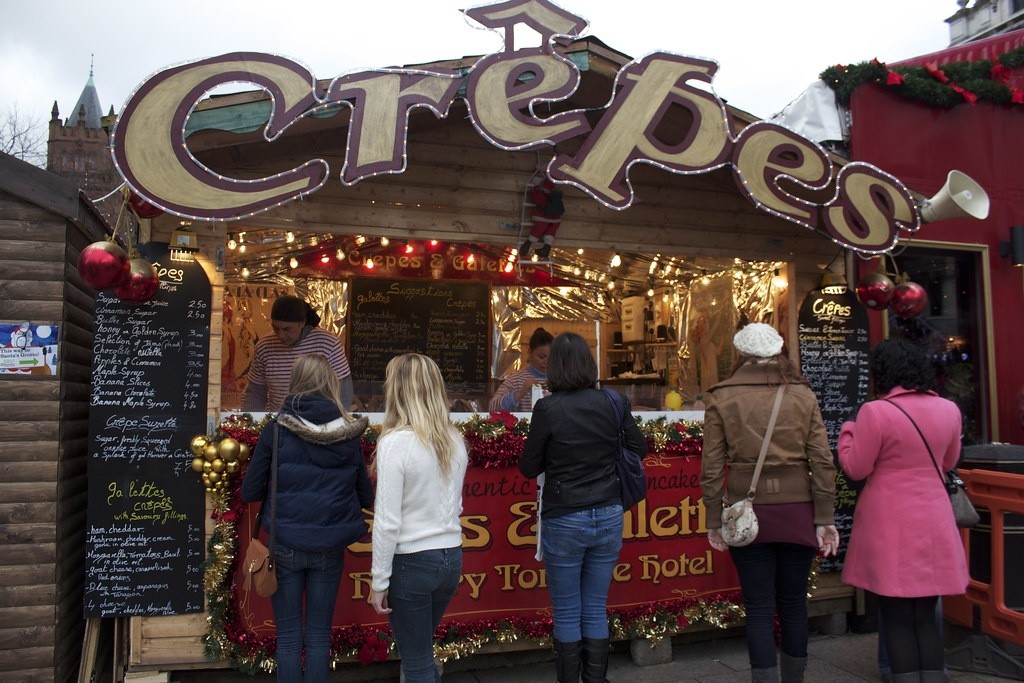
[957,440,1024,612]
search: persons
[368,353,469,683]
[486,327,555,413]
[518,332,650,683]
[701,323,839,683]
[243,295,354,412]
[836,339,972,683]
[239,354,374,683]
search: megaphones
[918,170,989,225]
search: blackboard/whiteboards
[797,286,876,572]
[83,265,212,617]
[349,276,486,402]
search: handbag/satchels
[535,472,544,561]
[721,497,759,547]
[604,388,647,512]
[242,538,277,597]
[945,470,979,528]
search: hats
[271,295,306,322]
[733,322,784,357]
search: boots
[582,633,610,683]
[921,670,946,683]
[780,651,808,683]
[552,637,583,683]
[893,672,920,683]
[751,666,778,683]
